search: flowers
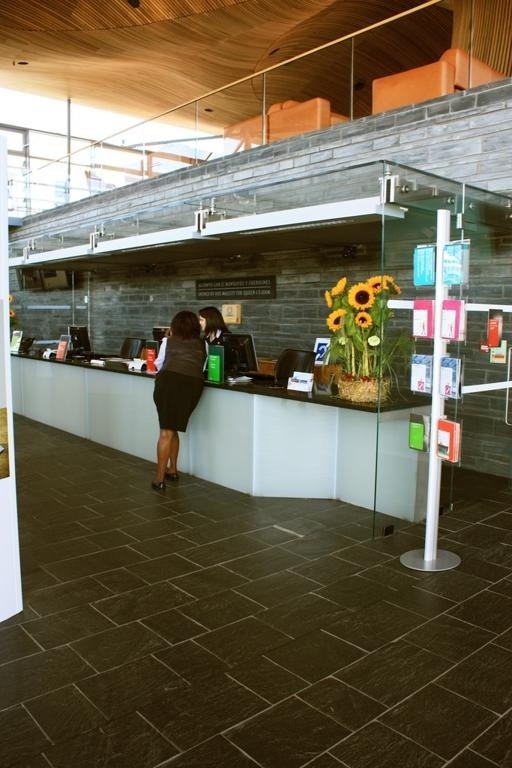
[321,275,416,379]
[9,294,17,319]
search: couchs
[224,97,347,150]
[370,47,508,116]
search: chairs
[273,347,318,385]
[119,337,148,359]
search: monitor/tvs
[39,268,70,291]
[222,333,259,382]
[68,326,91,352]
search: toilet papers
[90,359,105,365]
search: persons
[200,306,233,374]
[150,310,205,489]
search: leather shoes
[151,481,165,489]
[165,472,180,481]
